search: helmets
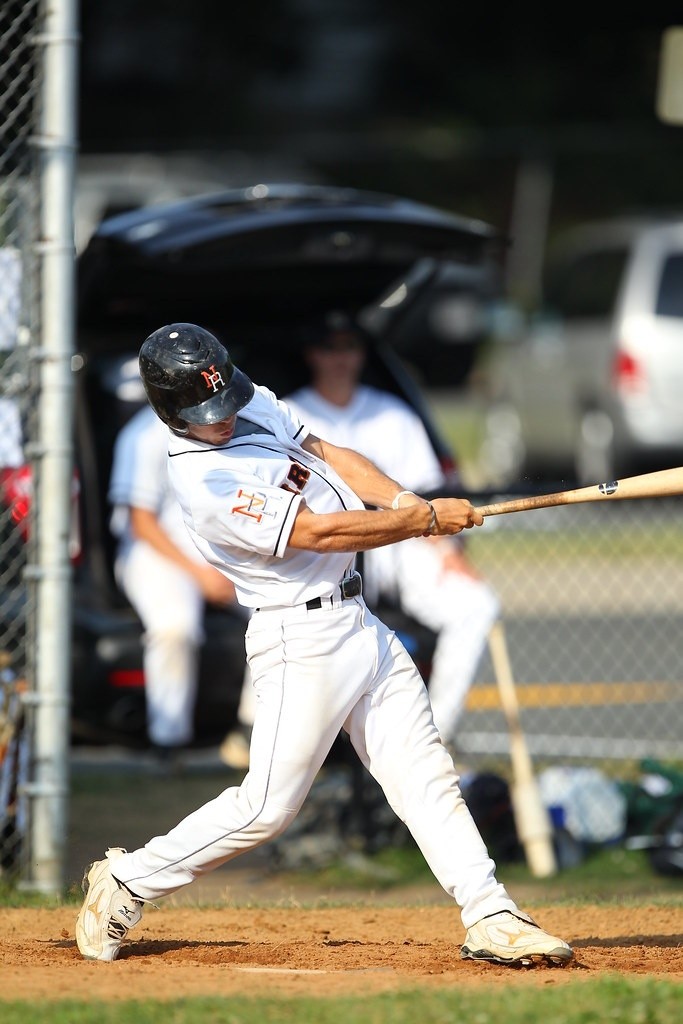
[139,323,255,437]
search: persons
[269,320,506,759]
[107,384,251,773]
[69,324,584,980]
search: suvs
[0,177,516,760]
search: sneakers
[459,911,573,967]
[75,847,144,962]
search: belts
[306,574,361,610]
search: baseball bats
[473,466,683,516]
[486,623,558,880]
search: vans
[491,217,683,498]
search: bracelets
[426,501,437,535]
[391,490,418,511]
